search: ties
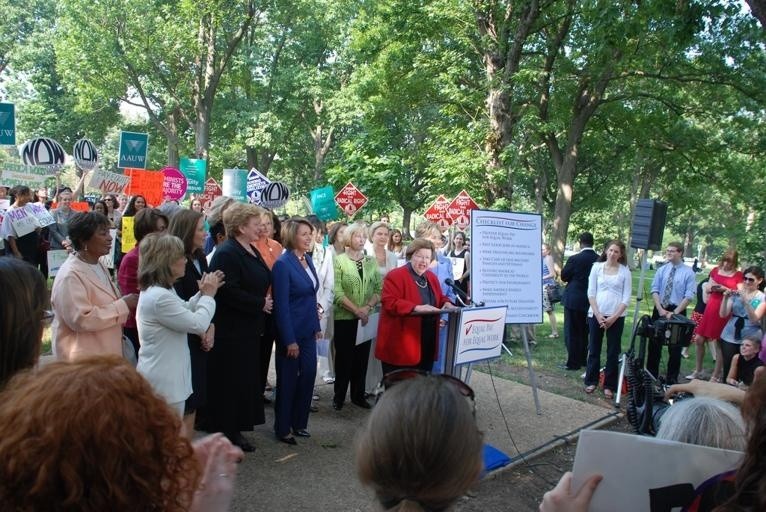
[662,267,676,309]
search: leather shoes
[293,430,311,437]
[263,398,272,404]
[332,402,343,410]
[685,370,702,380]
[371,392,376,397]
[365,391,371,399]
[323,376,333,383]
[558,365,568,369]
[265,384,272,390]
[709,376,721,383]
[232,436,256,452]
[281,434,297,446]
[310,404,319,412]
[681,352,690,359]
[312,395,320,400]
[603,389,614,399]
[585,385,596,393]
[351,400,371,409]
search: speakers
[630,199,668,250]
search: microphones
[445,279,469,300]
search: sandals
[544,333,559,338]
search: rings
[219,473,229,478]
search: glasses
[104,199,111,201]
[375,368,476,416]
[742,277,754,282]
[154,225,165,232]
[666,249,675,253]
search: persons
[538,371,765,512]
[652,379,756,455]
[0,183,473,454]
[529,243,560,339]
[511,323,537,344]
[557,232,600,370]
[681,248,766,390]
[646,242,697,384]
[356,369,484,512]
[584,240,632,399]
[1,353,243,512]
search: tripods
[580,252,658,408]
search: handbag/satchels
[123,335,138,367]
[546,283,566,303]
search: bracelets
[594,312,600,316]
[672,310,675,315]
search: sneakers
[509,337,517,343]
[528,340,537,345]
